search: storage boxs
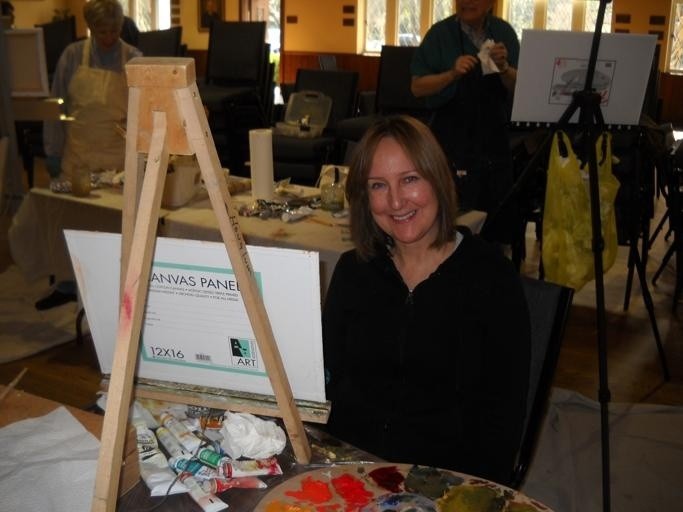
[0,380,140,505]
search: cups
[320,172,345,211]
[72,170,91,198]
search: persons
[409,1,519,207]
[41,0,143,192]
[320,113,531,490]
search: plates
[252,462,556,512]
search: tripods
[623,94,683,385]
[501,86,674,512]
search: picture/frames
[196,1,226,35]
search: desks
[0,360,385,512]
[30,164,488,305]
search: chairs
[494,272,576,490]
[0,20,683,259]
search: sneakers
[34,280,78,312]
